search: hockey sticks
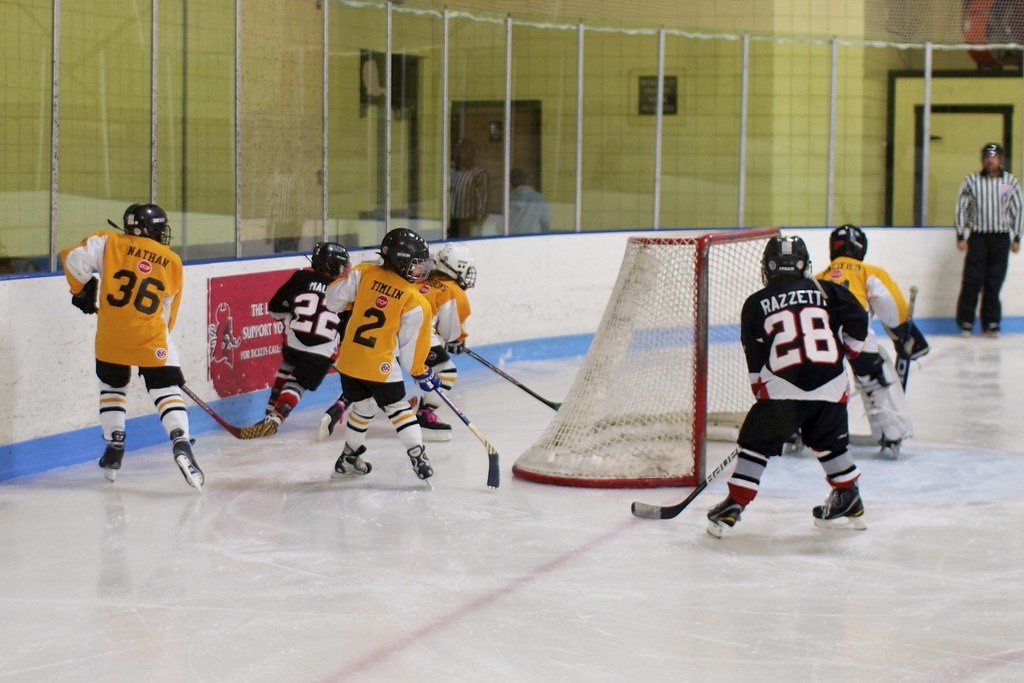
[434,388,500,489]
[848,285,918,446]
[631,446,742,519]
[93,305,278,441]
[463,347,589,420]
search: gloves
[446,340,465,355]
[70,276,99,314]
[412,369,443,391]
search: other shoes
[881,441,900,459]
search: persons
[499,169,551,236]
[448,139,488,238]
[267,149,307,251]
[953,141,1024,337]
[63,202,204,493]
[783,224,914,459]
[707,236,868,537]
[259,239,478,442]
[326,226,434,486]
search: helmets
[829,225,868,261]
[761,236,809,286]
[436,242,477,290]
[311,242,350,278]
[381,229,431,283]
[454,139,476,155]
[982,143,1001,154]
[123,203,172,245]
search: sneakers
[813,486,868,530]
[705,497,746,538]
[99,430,126,481]
[331,442,372,478]
[318,395,349,439]
[407,444,434,488]
[961,322,973,336]
[987,321,1000,338]
[170,428,205,493]
[415,396,452,441]
[253,403,293,434]
